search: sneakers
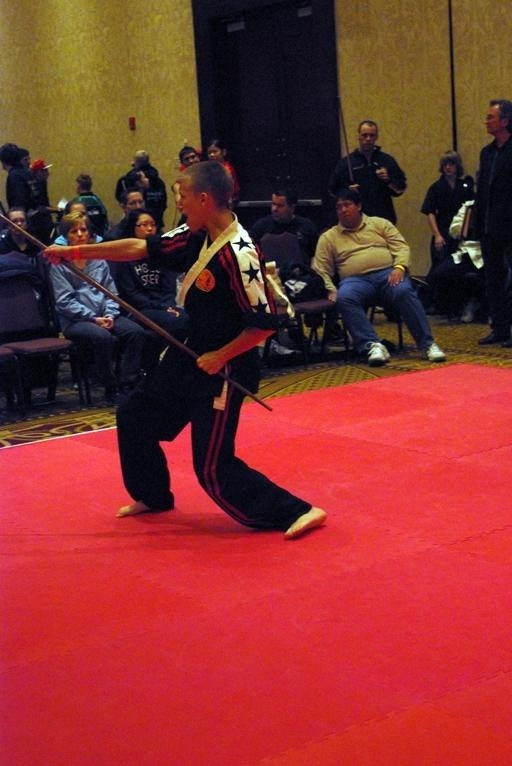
[266,338,303,358]
[322,322,347,346]
[104,388,131,406]
[365,341,391,366]
[459,296,481,325]
[419,342,447,363]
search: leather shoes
[477,326,509,344]
[501,334,512,348]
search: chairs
[259,229,350,370]
[0,264,94,422]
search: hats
[29,157,55,171]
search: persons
[249,99,510,368]
[0,138,240,407]
[43,159,328,539]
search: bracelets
[70,246,84,266]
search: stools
[368,301,409,353]
[0,345,21,426]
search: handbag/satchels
[276,259,331,305]
